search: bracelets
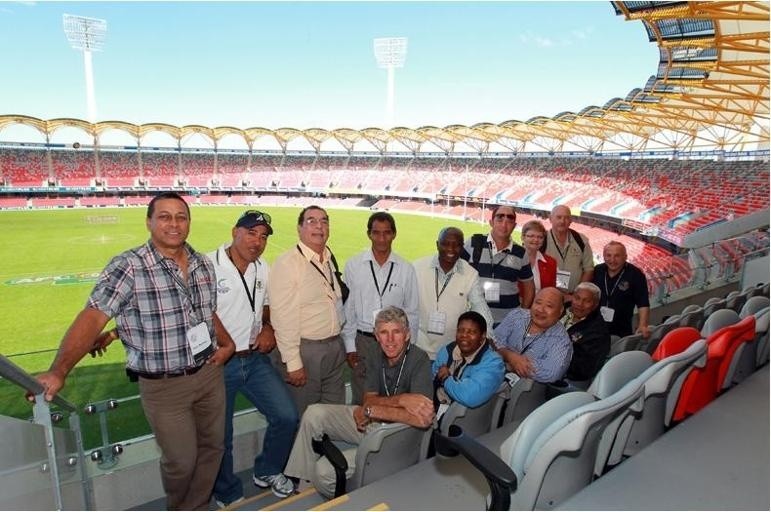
[109,331,118,340]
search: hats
[235,213,274,234]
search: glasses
[494,213,515,220]
[305,219,328,225]
[243,209,271,224]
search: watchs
[364,405,371,417]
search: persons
[412,227,495,362]
[593,241,651,341]
[518,220,557,306]
[88,212,299,508]
[560,281,611,382]
[25,193,236,511]
[283,305,433,498]
[460,205,535,328]
[342,212,420,407]
[493,287,573,384]
[266,205,346,415]
[545,204,594,301]
[433,311,506,410]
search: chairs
[277,155,769,277]
[545,328,651,393]
[701,308,741,340]
[744,285,761,300]
[653,328,698,361]
[653,315,755,426]
[681,303,703,326]
[312,423,433,500]
[704,297,724,315]
[440,386,510,444]
[589,338,707,458]
[611,331,647,352]
[739,296,770,367]
[665,312,685,327]
[726,291,747,311]
[429,378,645,512]
[502,377,548,425]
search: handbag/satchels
[326,245,350,306]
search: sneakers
[253,472,295,498]
[216,497,245,510]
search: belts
[138,359,205,380]
